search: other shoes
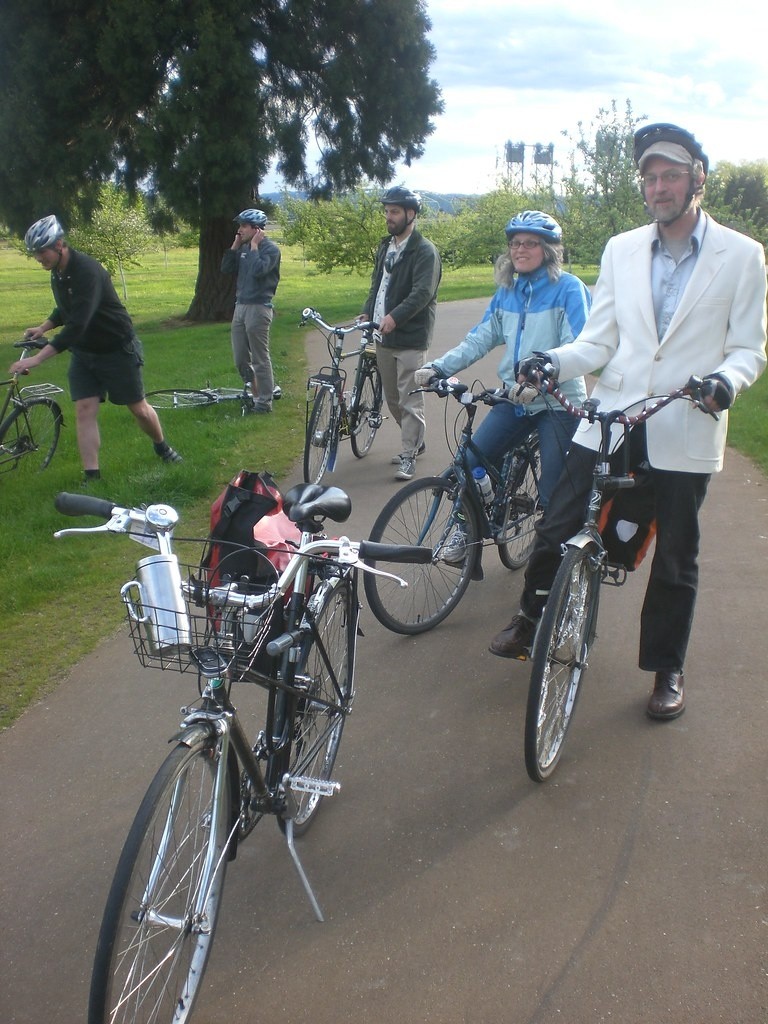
[249,398,272,415]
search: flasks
[120,554,193,655]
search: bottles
[472,466,495,505]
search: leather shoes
[647,668,686,719]
[491,614,536,658]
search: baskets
[120,562,278,681]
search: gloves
[508,382,538,405]
[415,369,437,388]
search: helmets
[25,215,63,251]
[233,209,267,230]
[634,123,708,184]
[505,210,563,244]
[379,187,420,211]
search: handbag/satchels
[206,470,314,682]
[597,449,657,571]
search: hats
[637,142,692,171]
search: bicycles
[515,360,721,782]
[145,380,283,416]
[55,483,433,1023]
[361,371,544,636]
[0,335,67,477]
[297,307,389,484]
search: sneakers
[395,456,416,479]
[393,441,425,463]
[161,447,183,466]
[441,513,470,562]
[79,478,101,489]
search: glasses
[385,251,396,274]
[508,239,541,249]
[641,168,689,186]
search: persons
[415,210,592,562]
[220,209,281,416]
[490,122,768,719]
[354,186,442,479]
[8,215,182,489]
